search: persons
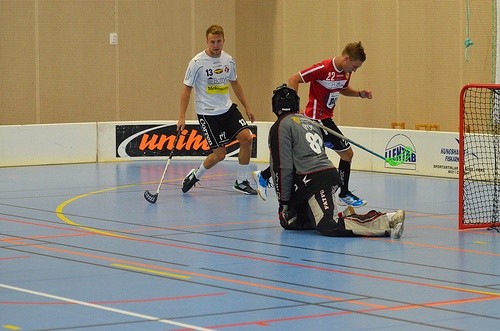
[267,84,406,239]
[176,25,258,195]
[252,41,373,208]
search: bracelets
[358,90,364,98]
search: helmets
[271,88,299,115]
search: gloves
[279,201,288,227]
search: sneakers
[336,191,368,208]
[180,168,201,193]
[343,205,356,218]
[387,210,406,239]
[231,178,258,196]
[252,169,274,200]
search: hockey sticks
[315,123,413,166]
[144,127,182,204]
